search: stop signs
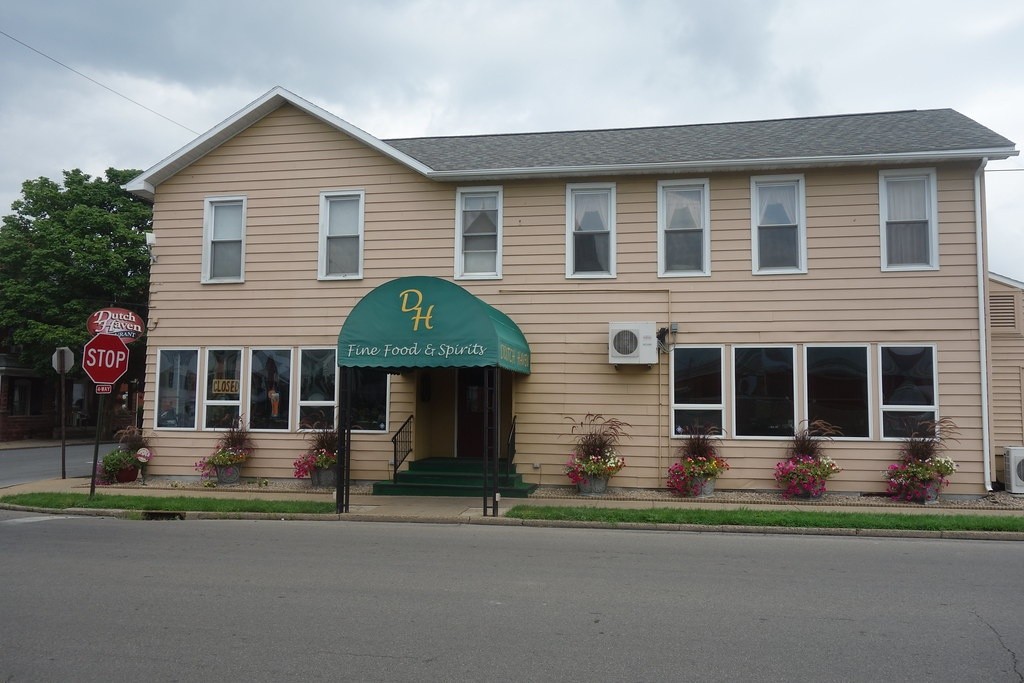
[82,333,129,385]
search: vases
[916,477,941,506]
[215,463,242,484]
[575,472,609,494]
[114,467,138,483]
[797,481,826,499]
[309,463,337,486]
[695,476,716,499]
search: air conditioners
[608,321,658,366]
[1003,447,1024,495]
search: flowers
[883,456,959,501]
[293,449,337,479]
[773,455,843,500]
[195,442,249,479]
[94,447,153,485]
[560,451,626,485]
[666,453,729,497]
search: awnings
[335,276,530,515]
